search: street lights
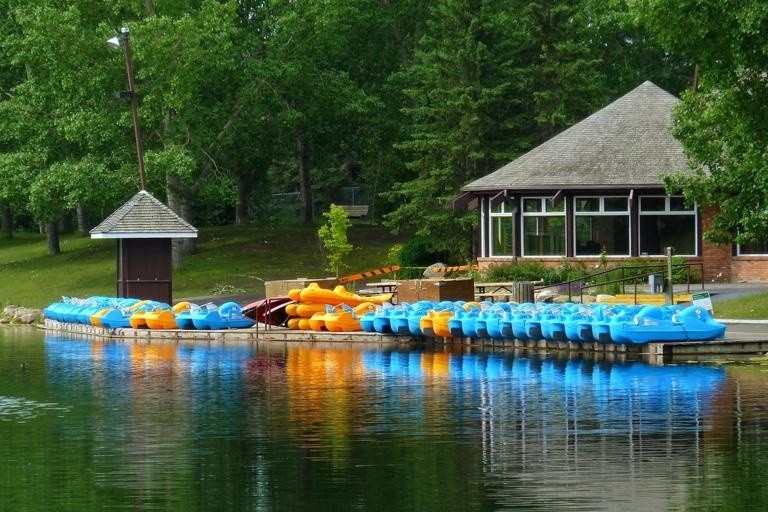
[106,28,148,189]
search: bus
[272,186,369,217]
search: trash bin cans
[513,281,534,303]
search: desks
[366,281,397,305]
[476,280,543,303]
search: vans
[390,278,474,305]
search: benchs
[357,288,397,306]
[474,290,541,304]
